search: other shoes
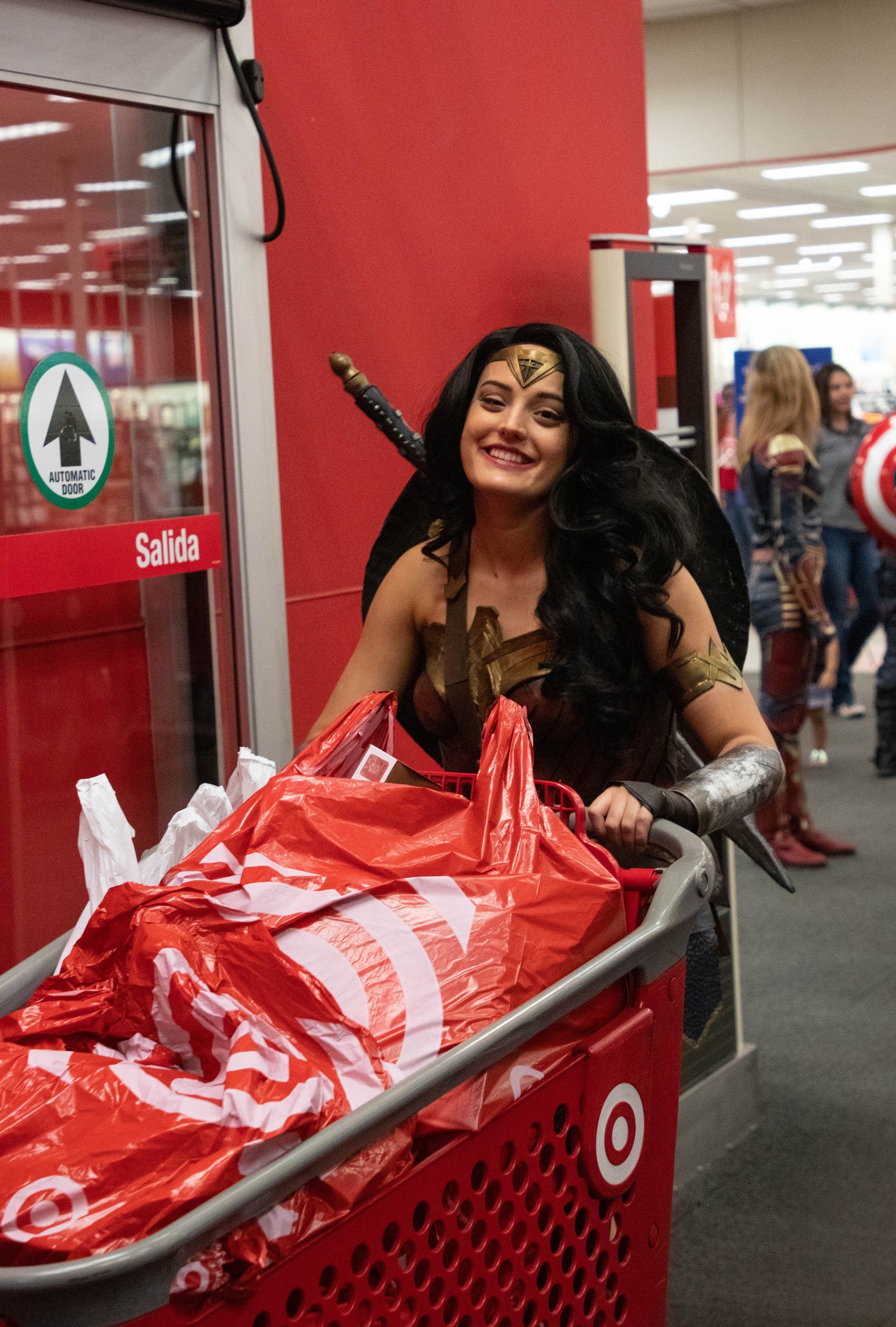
[836,702,865,720]
[809,749,827,769]
[872,750,896,776]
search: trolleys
[0,811,718,1326]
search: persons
[291,322,786,1051]
[738,347,858,869]
[716,359,896,778]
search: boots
[754,796,827,869]
[784,758,857,857]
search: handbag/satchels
[54,745,275,972]
[166,686,627,1130]
